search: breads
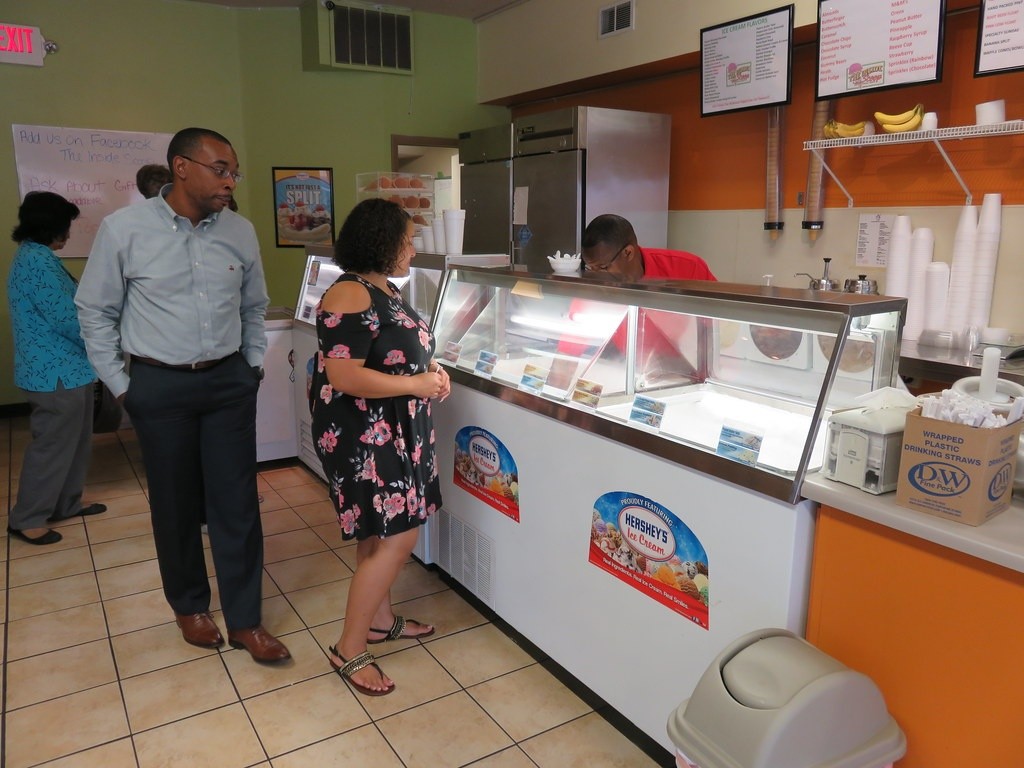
[361,177,432,227]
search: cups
[883,191,1002,348]
[411,208,469,254]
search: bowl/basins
[549,257,581,276]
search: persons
[6,191,108,545]
[74,126,293,664]
[310,197,451,695]
[556,214,740,382]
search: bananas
[822,118,866,140]
[874,103,924,133]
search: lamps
[0,22,59,66]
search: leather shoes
[174,610,225,649]
[226,624,291,665]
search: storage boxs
[895,405,1023,526]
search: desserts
[278,201,331,243]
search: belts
[128,353,220,372]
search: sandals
[330,645,395,697]
[367,616,435,644]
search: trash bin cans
[666,628,908,768]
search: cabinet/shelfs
[356,172,436,230]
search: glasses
[178,154,245,185]
[585,243,630,273]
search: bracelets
[430,363,438,364]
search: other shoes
[6,523,62,545]
[67,503,107,518]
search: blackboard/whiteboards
[10,123,175,257]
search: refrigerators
[457,105,670,287]
[254,307,296,465]
